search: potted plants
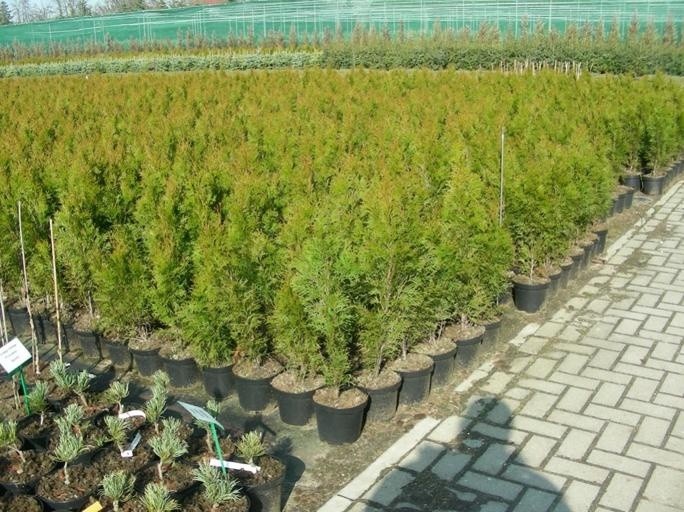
[1,361,287,511]
[1,103,683,446]
